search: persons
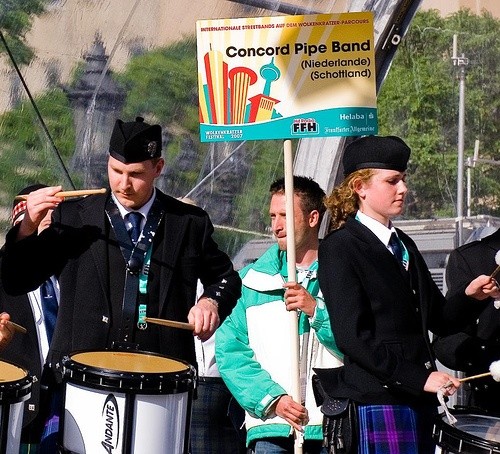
[434,229,500,417]
[0,184,61,378]
[215,176,344,454]
[13,117,243,454]
[317,136,500,454]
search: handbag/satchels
[320,396,360,454]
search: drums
[0,358,39,454]
[41,347,197,454]
[429,409,500,454]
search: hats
[109,116,163,164]
[343,136,410,178]
[12,185,65,226]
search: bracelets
[207,298,218,307]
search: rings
[210,323,215,327]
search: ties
[389,233,402,265]
[125,212,143,244]
[40,279,58,348]
